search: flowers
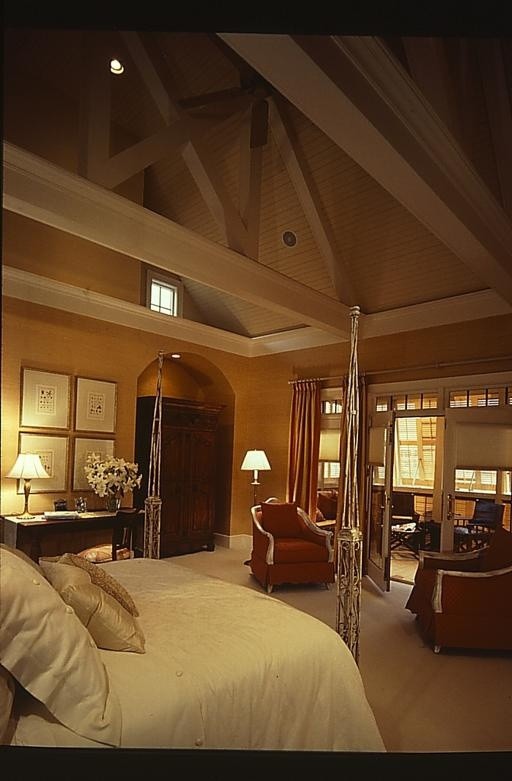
[83,450,143,510]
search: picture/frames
[19,364,74,431]
[15,430,70,496]
[70,433,117,493]
[72,374,120,435]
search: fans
[174,33,279,150]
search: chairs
[403,525,512,657]
[452,497,505,554]
[73,510,137,568]
[241,495,337,596]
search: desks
[1,510,135,566]
[376,523,430,561]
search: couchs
[311,490,420,532]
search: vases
[107,491,123,509]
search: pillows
[259,501,303,540]
[475,525,512,573]
[38,557,150,655]
[0,540,51,585]
[36,549,143,619]
[314,504,328,522]
[317,490,346,521]
[0,547,125,748]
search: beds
[0,539,391,754]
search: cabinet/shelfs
[135,394,226,552]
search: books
[44,511,80,520]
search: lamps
[239,448,272,507]
[3,452,55,520]
